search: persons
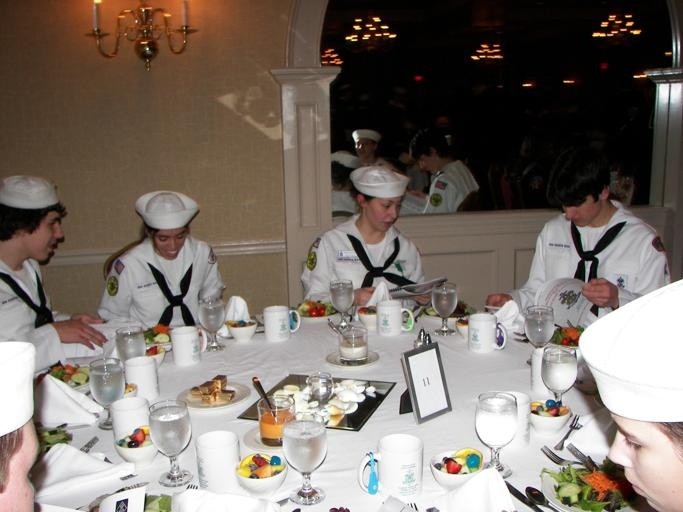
[96,189,225,328]
[0,176,108,378]
[331,127,479,214]
[486,157,671,311]
[1,342,42,512]
[300,166,430,306]
[579,279,681,512]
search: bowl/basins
[529,402,571,431]
[232,450,289,494]
[228,320,256,342]
[455,324,467,340]
[428,449,483,487]
[359,312,377,330]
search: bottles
[414,329,429,350]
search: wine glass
[540,345,576,402]
[88,291,240,487]
[525,305,554,365]
[473,389,517,482]
[283,411,328,506]
[433,280,458,336]
[329,279,355,333]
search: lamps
[87,2,196,72]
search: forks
[539,443,595,470]
[554,414,585,451]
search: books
[386,274,449,301]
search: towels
[216,296,250,338]
[28,442,136,512]
[364,280,391,306]
[32,374,106,428]
[494,299,526,333]
[424,466,517,512]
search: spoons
[525,487,561,511]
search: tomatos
[318,308,325,316]
[309,308,319,317]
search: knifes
[503,479,543,511]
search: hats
[0,340,35,436]
[351,129,381,143]
[349,166,411,199]
[134,190,199,230]
[331,150,359,169]
[0,175,58,210]
[577,278,682,424]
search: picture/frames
[398,341,452,424]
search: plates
[177,381,250,411]
[327,350,380,367]
[242,424,283,454]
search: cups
[306,371,332,403]
[376,299,414,335]
[338,328,369,361]
[467,312,506,352]
[355,431,423,499]
[258,393,294,445]
[499,391,531,448]
[529,348,552,396]
[261,304,300,341]
